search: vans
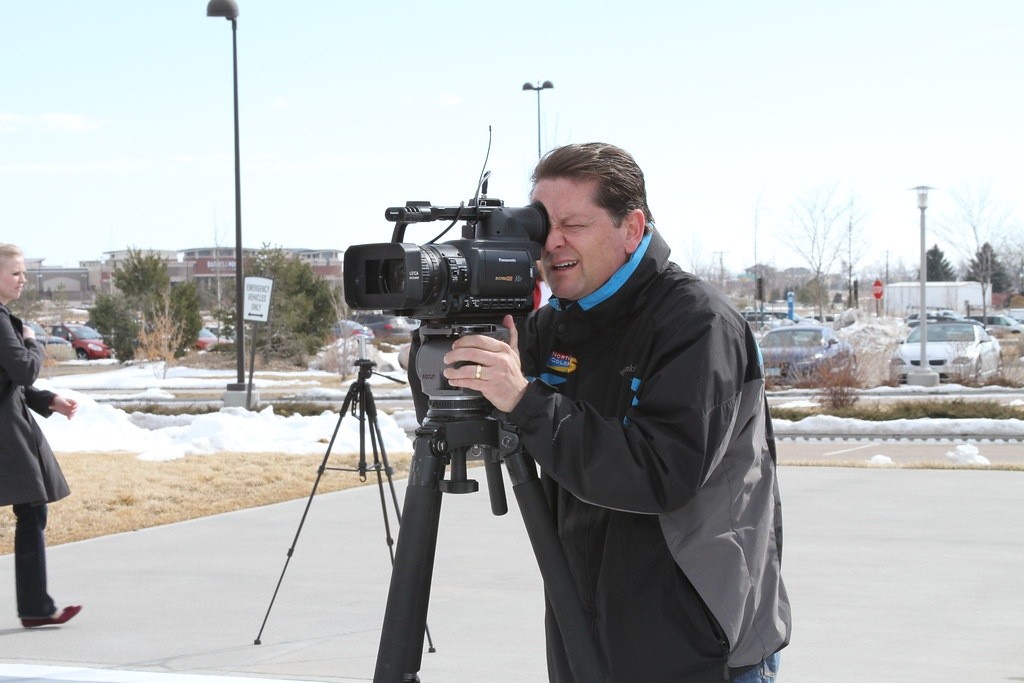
[1003,309,1024,322]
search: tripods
[254,359,436,653]
[373,321,608,683]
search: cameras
[357,336,367,359]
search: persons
[443,144,792,683]
[0,243,83,628]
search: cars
[348,313,412,343]
[333,319,375,343]
[46,324,113,360]
[20,319,72,359]
[904,310,984,330]
[741,310,822,325]
[192,326,251,351]
[759,325,858,385]
[890,322,1004,389]
[966,314,1024,333]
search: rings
[476,365,481,379]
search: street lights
[206,0,261,410]
[521,80,553,160]
[906,186,939,386]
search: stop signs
[872,280,883,299]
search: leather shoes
[21,605,81,628]
